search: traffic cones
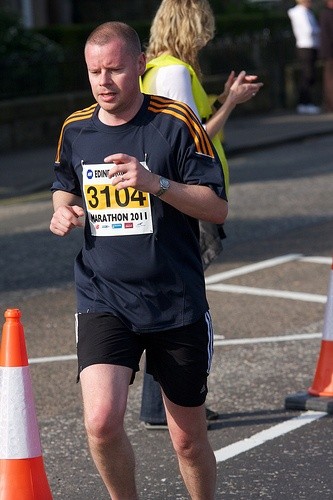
[284,257,333,412]
[0,308,67,500]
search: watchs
[153,175,171,197]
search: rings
[252,92,256,96]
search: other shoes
[206,408,218,420]
[143,421,168,429]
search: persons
[320,0,333,113]
[137,1,266,430]
[50,23,229,500]
[286,0,323,114]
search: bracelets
[213,100,223,110]
